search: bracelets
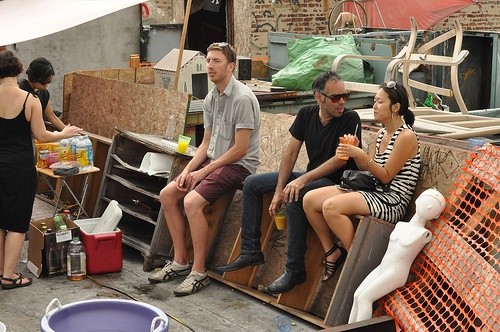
[367,158,372,165]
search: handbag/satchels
[340,170,402,206]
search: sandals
[1,273,32,290]
[0,272,23,284]
[320,244,348,282]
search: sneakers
[147,260,191,283]
[174,271,211,295]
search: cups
[47,153,61,168]
[273,208,287,230]
[176,134,192,154]
[338,137,355,161]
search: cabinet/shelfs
[93,127,198,270]
[190,182,393,330]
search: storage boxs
[24,215,79,278]
[75,219,123,273]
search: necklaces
[1,83,17,87]
[386,132,392,140]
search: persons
[303,81,421,281]
[348,188,446,323]
[0,50,83,289]
[19,56,86,241]
[148,42,261,295]
[216,71,362,294]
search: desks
[31,162,99,220]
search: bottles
[62,209,74,222]
[60,133,94,171]
[66,237,87,281]
[39,222,52,233]
[57,225,67,233]
[166,114,176,139]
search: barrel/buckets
[39,298,171,332]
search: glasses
[319,90,350,103]
[385,81,396,90]
[207,43,233,63]
[41,80,51,85]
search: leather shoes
[217,253,265,271]
[265,270,306,293]
